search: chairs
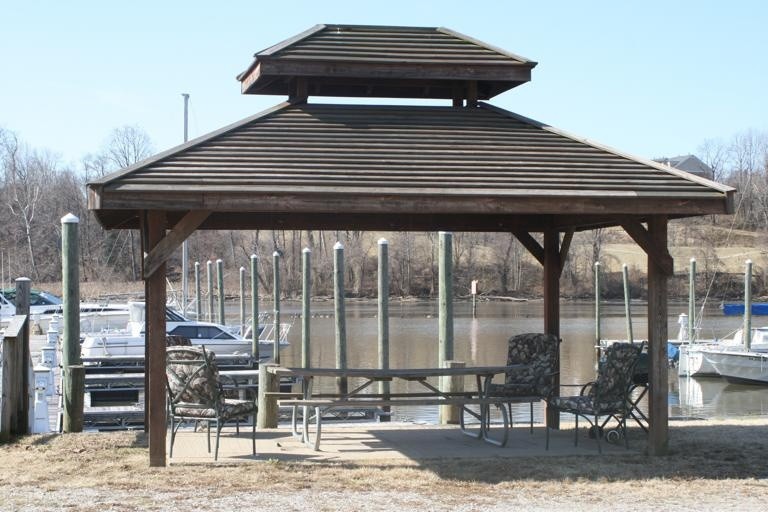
[545,340,646,454]
[482,332,562,433]
[166,334,240,434]
[165,345,258,461]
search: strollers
[589,342,678,444]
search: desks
[266,366,529,449]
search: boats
[596,339,767,368]
[721,303,767,316]
[77,299,293,364]
[0,286,63,318]
[746,344,767,399]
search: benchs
[263,391,486,435]
[276,395,540,449]
